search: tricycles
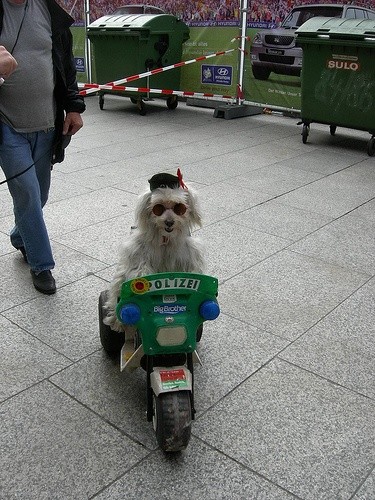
[97,272,221,453]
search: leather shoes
[30,268,56,294]
[12,243,29,265]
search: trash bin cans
[85,14,190,116]
[294,16,374,157]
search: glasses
[151,202,189,216]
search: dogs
[104,173,206,364]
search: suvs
[250,4,375,79]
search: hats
[148,168,188,192]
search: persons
[0,0,86,294]
[57,0,375,23]
[0,45,18,80]
